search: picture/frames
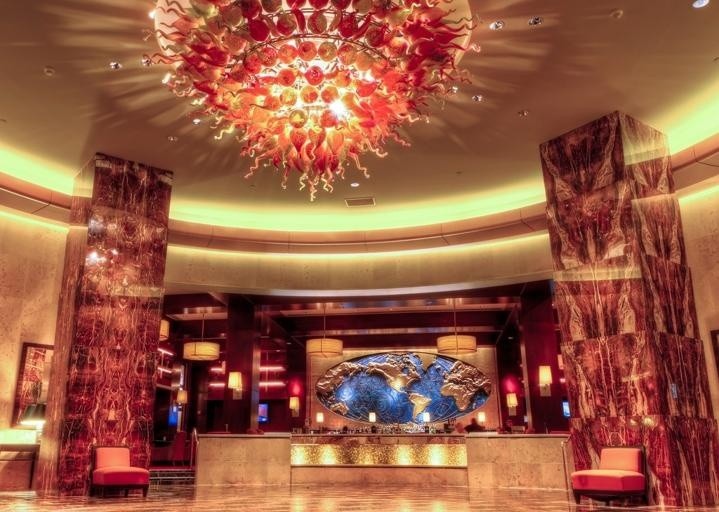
[10,341,54,430]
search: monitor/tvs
[258,402,269,423]
[562,397,570,418]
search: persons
[465,417,483,432]
[501,417,513,433]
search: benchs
[289,443,469,492]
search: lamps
[141,0,483,200]
[436,297,477,355]
[368,411,376,422]
[306,301,343,359]
[159,320,170,341]
[507,393,518,407]
[19,404,47,444]
[423,412,429,422]
[316,412,324,422]
[183,293,220,361]
[176,389,187,403]
[478,412,486,422]
[539,366,552,386]
[289,396,300,410]
[228,372,242,388]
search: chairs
[90,446,150,498]
[569,445,649,507]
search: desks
[0,445,40,489]
